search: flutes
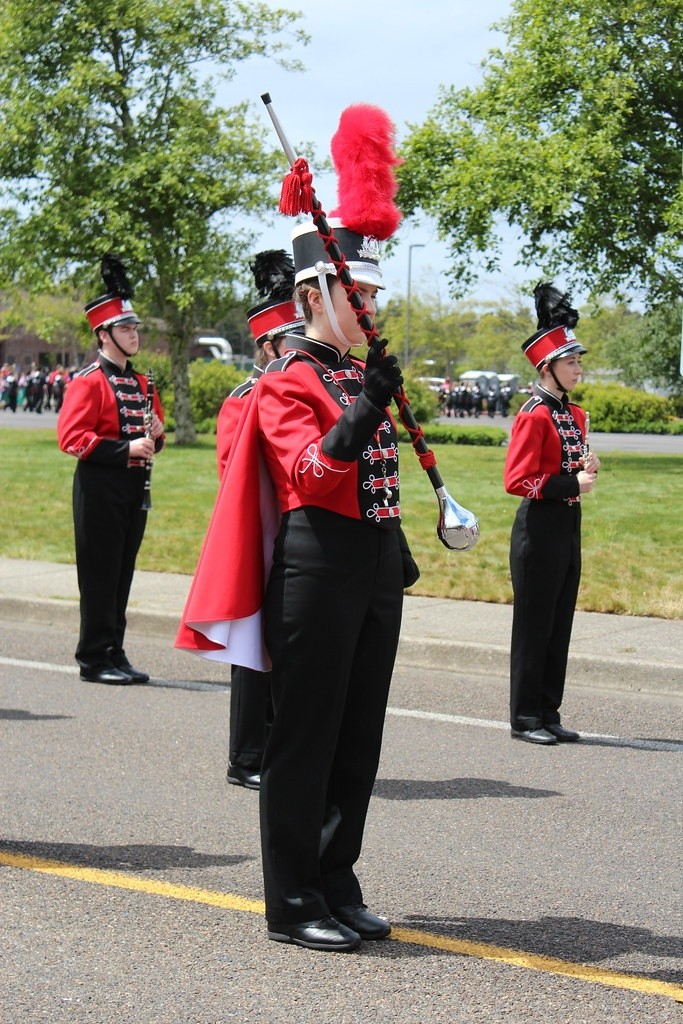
[583,412,590,465]
[141,366,155,513]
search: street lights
[406,245,427,363]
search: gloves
[364,338,404,409]
[402,549,421,589]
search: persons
[171,227,419,950]
[502,280,600,743]
[58,248,167,684]
[430,377,513,418]
[0,361,79,413]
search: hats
[521,280,588,372]
[246,249,306,348]
[292,103,397,290]
[84,252,141,333]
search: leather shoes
[510,724,579,743]
[267,901,392,949]
[226,760,260,789]
[79,664,149,684]
